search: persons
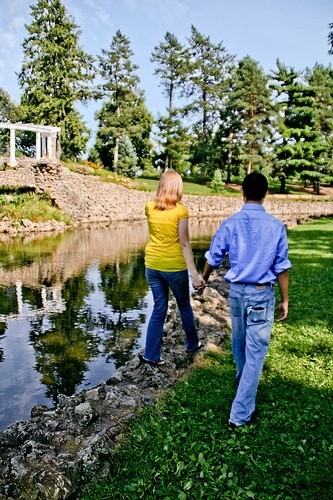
[137,169,207,368]
[191,171,293,431]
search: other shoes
[185,341,205,353]
[140,356,166,366]
